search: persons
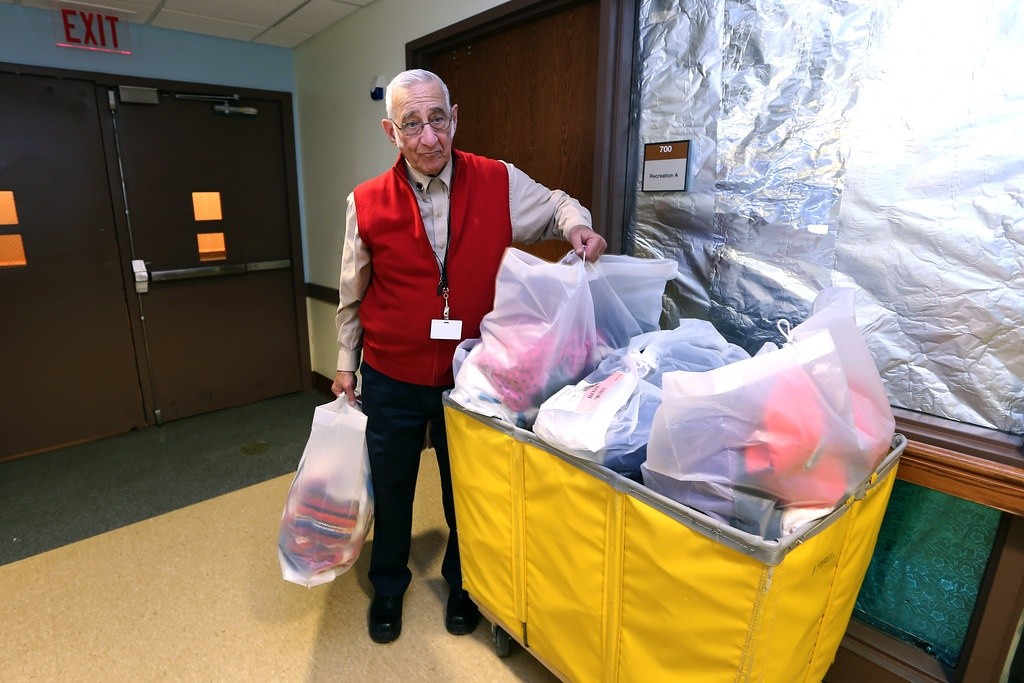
[332,70,606,644]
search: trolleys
[440,388,908,683]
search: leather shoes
[367,566,413,644]
[444,585,481,636]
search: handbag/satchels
[532,319,753,472]
[276,391,374,591]
[448,245,679,431]
[639,286,896,541]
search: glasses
[389,108,452,137]
